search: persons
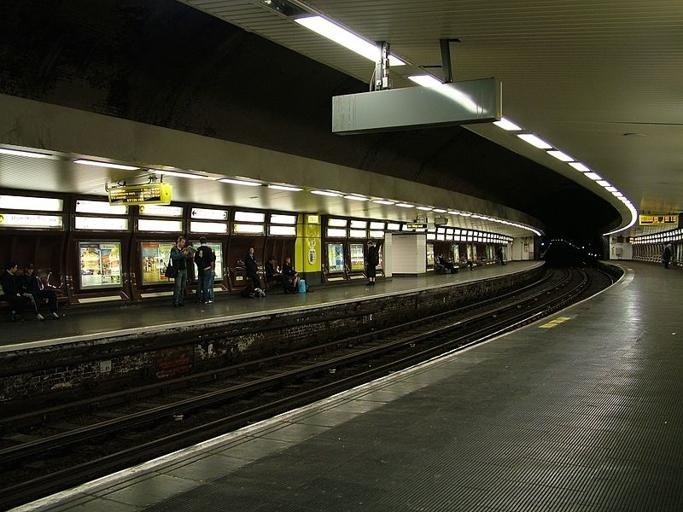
[433,252,458,274]
[498,247,506,265]
[461,253,474,271]
[245,246,261,298]
[661,243,672,269]
[194,237,216,305]
[264,256,315,294]
[0,262,29,324]
[170,235,194,308]
[364,240,379,286]
[17,263,60,322]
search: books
[181,246,199,257]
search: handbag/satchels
[241,284,255,298]
[284,286,297,294]
[165,266,178,277]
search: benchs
[2,270,72,323]
